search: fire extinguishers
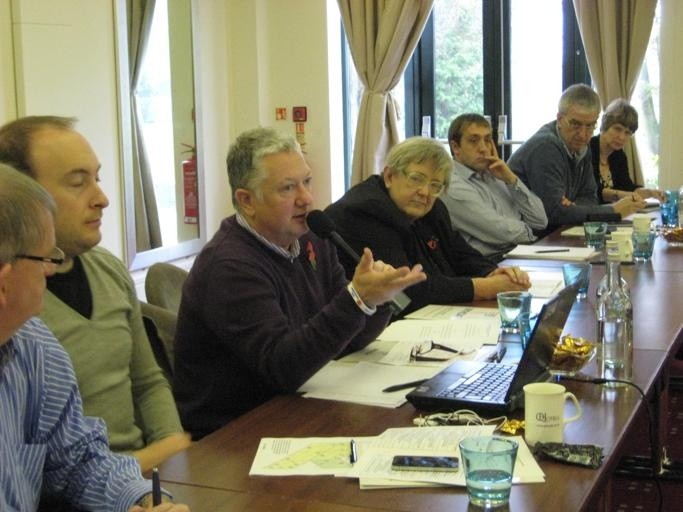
[180,142,199,224]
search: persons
[322,137,533,322]
[589,98,670,204]
[438,113,549,265]
[507,83,647,240]
[0,163,191,512]
[172,126,429,442]
[0,113,196,476]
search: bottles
[593,240,635,388]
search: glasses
[398,165,445,201]
[11,247,66,275]
[407,336,476,369]
[562,113,598,133]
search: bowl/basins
[541,335,596,377]
[661,228,682,247]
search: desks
[141,202,682,512]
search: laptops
[405,278,582,417]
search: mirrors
[113,1,206,271]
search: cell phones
[391,455,458,473]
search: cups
[657,187,679,226]
[516,311,539,349]
[497,289,533,334]
[633,213,653,231]
[457,435,520,509]
[561,262,591,299]
[582,220,608,249]
[519,383,582,444]
[611,231,634,264]
[632,229,656,262]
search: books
[502,244,596,261]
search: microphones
[306,208,360,265]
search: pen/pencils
[382,379,429,392]
[536,249,569,253]
[152,467,161,507]
[632,195,635,202]
[590,261,635,265]
[349,440,357,467]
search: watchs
[610,189,618,202]
